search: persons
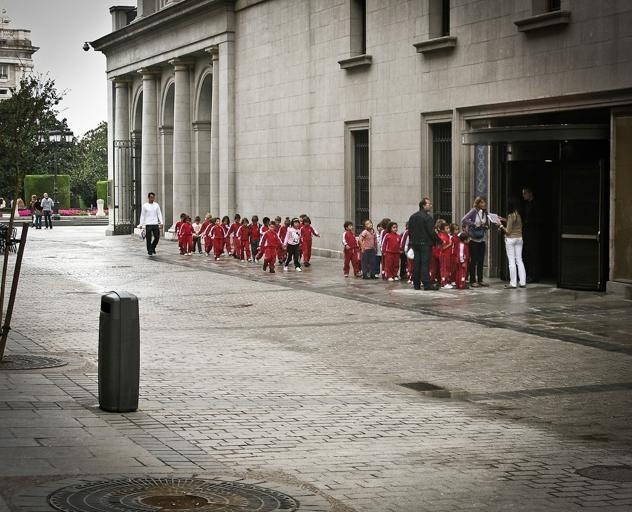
[174,213,321,273]
[28,195,37,228]
[140,192,164,260]
[495,195,527,289]
[40,193,54,229]
[33,197,43,229]
[342,216,472,290]
[407,197,446,290]
[520,184,547,284]
[462,196,490,288]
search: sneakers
[503,283,518,289]
[343,272,470,290]
[471,282,481,288]
[478,281,489,287]
[519,285,527,288]
[148,250,156,255]
[178,246,311,273]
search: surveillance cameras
[83,44,89,51]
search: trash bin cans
[98,289,140,412]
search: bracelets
[498,225,503,231]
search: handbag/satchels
[467,208,488,240]
